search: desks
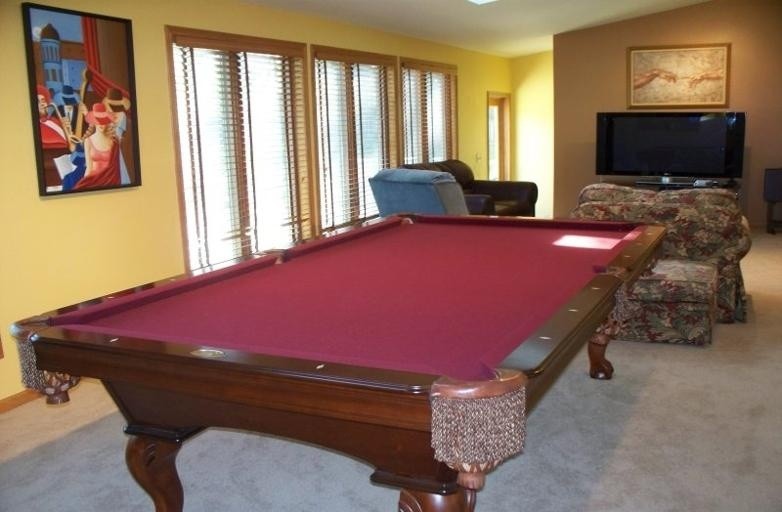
[8,212,666,512]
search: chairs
[403,160,537,216]
[369,168,469,219]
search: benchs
[608,259,717,346]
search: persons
[35,83,134,192]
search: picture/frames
[626,43,731,109]
[20,3,142,197]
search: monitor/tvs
[595,111,746,185]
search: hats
[53,85,79,106]
[102,88,131,113]
[86,103,116,125]
[36,84,51,104]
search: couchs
[579,183,749,230]
[569,201,753,322]
